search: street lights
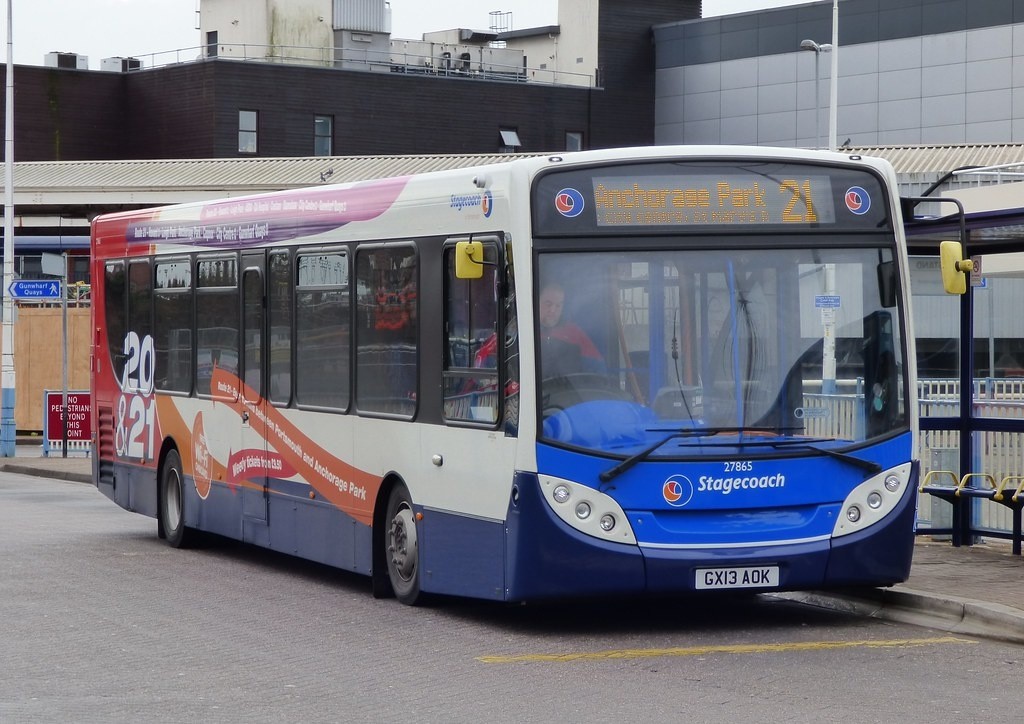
[800,39,839,152]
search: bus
[90,144,921,614]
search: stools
[919,471,996,547]
[989,476,1024,555]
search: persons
[473,278,608,379]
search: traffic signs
[8,280,61,298]
